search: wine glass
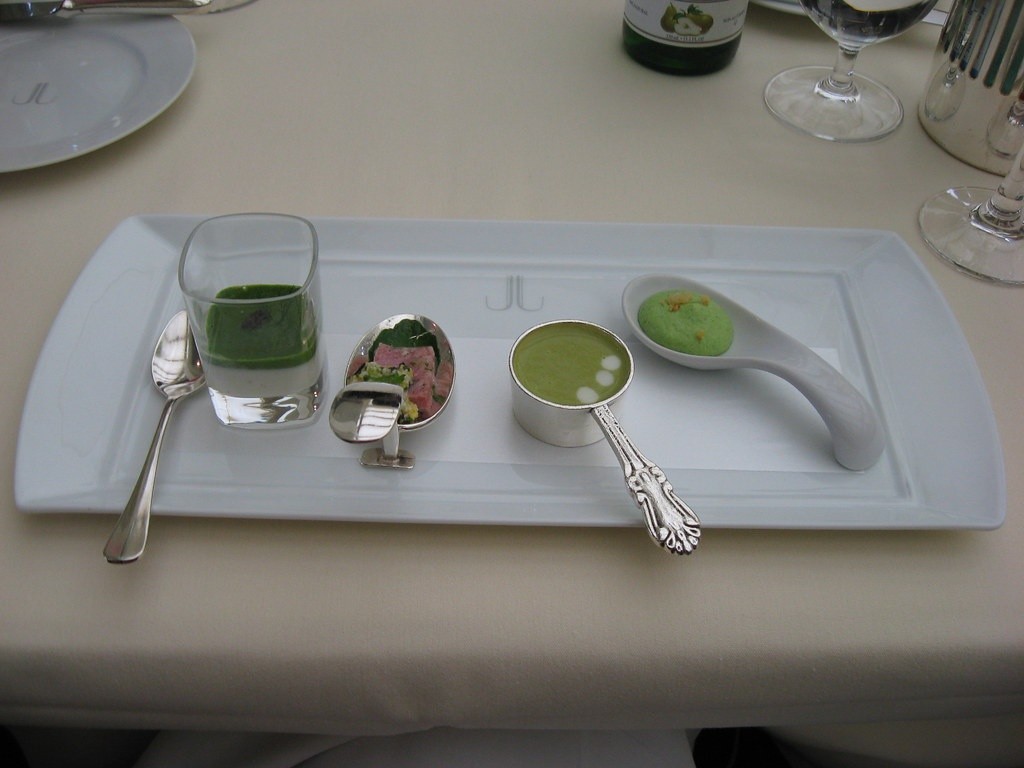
[764,0,937,143]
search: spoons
[102,308,210,564]
[621,274,886,470]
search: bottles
[622,0,749,76]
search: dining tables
[1,0,1024,735]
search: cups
[179,212,331,431]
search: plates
[0,7,197,173]
[10,216,1008,531]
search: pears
[661,2,713,36]
